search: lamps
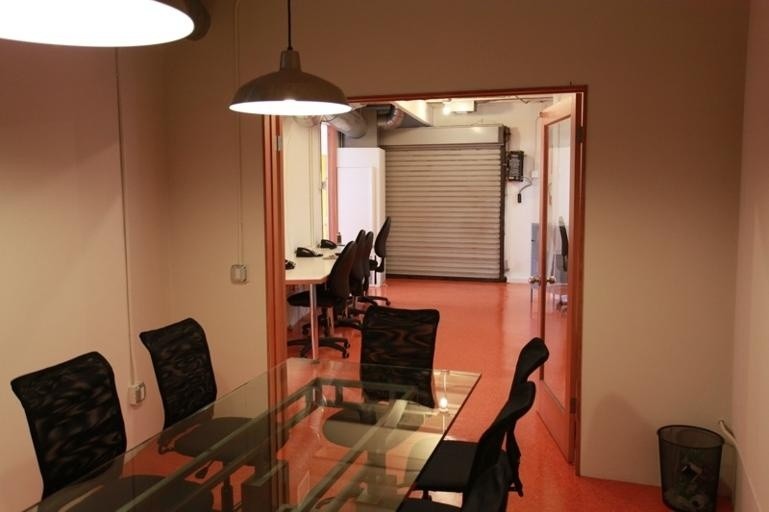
[230,0,352,117]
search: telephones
[320,239,337,249]
[284,259,295,270]
[296,247,315,257]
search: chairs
[285,216,393,359]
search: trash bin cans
[657,424,724,512]
